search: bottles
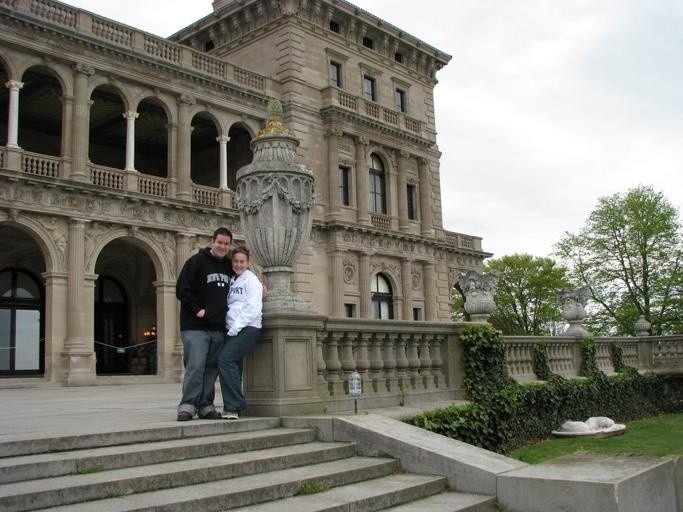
[348,368,361,397]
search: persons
[215,245,263,420]
[174,226,268,421]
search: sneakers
[221,409,240,419]
[197,410,221,419]
[177,411,198,421]
